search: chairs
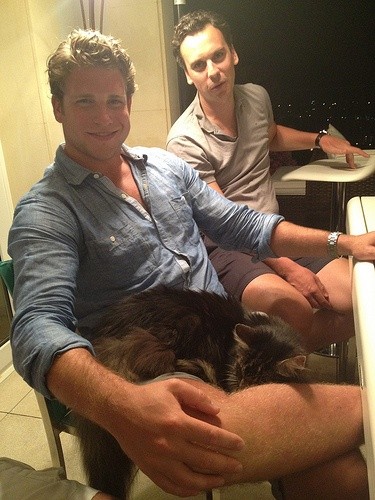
[1,259,106,486]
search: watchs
[315,129,331,145]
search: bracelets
[327,232,344,259]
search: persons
[166,10,371,357]
[10,28,374,500]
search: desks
[282,147,375,236]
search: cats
[72,284,310,500]
